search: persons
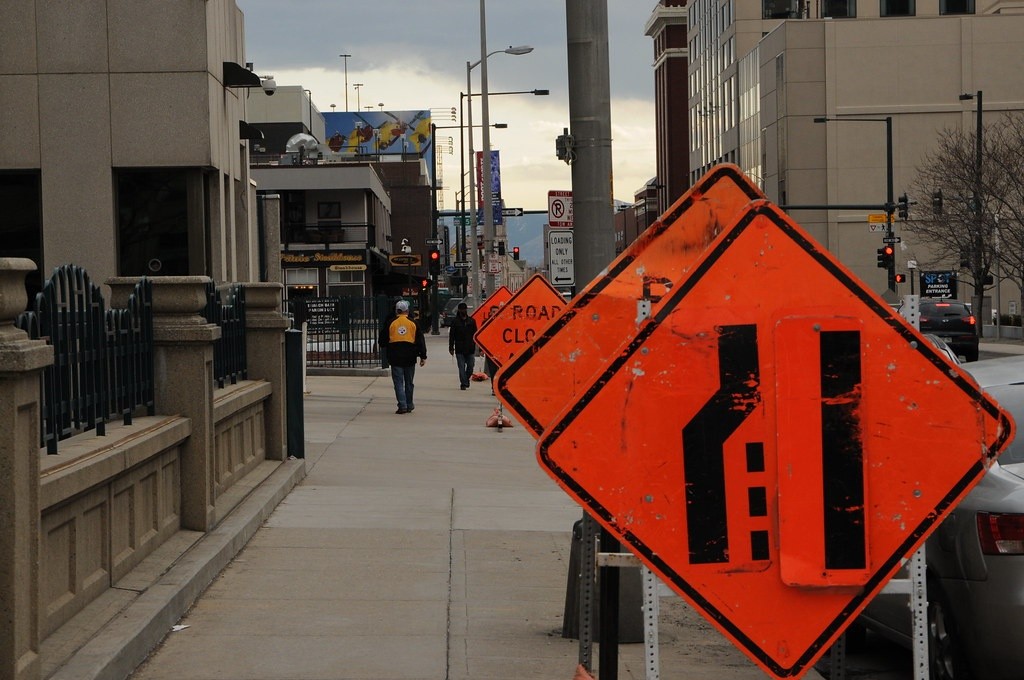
[378,300,427,413]
[449,303,477,390]
[482,305,500,395]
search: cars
[920,298,979,363]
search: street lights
[431,123,508,336]
[460,89,550,297]
[332,55,384,112]
[467,47,534,362]
[814,115,896,291]
[959,89,982,338]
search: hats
[458,303,468,310]
[395,300,410,314]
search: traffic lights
[896,274,906,284]
[884,245,894,269]
[877,247,885,269]
[898,195,908,219]
[514,247,520,260]
[422,279,432,287]
[429,250,440,274]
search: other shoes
[460,381,470,390]
[395,403,415,414]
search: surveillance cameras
[262,79,277,96]
